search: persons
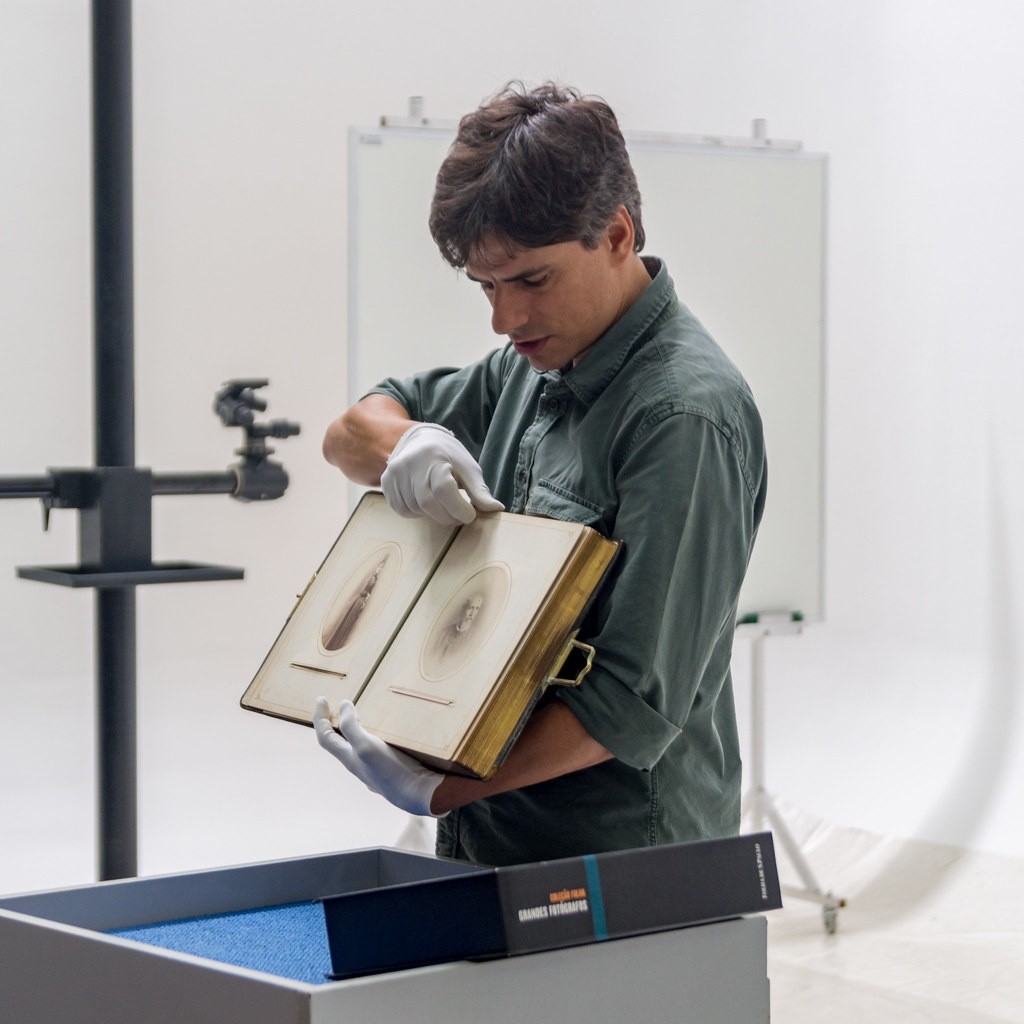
[320,84,768,869]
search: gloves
[313,695,452,819]
[381,423,505,529]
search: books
[237,489,631,782]
